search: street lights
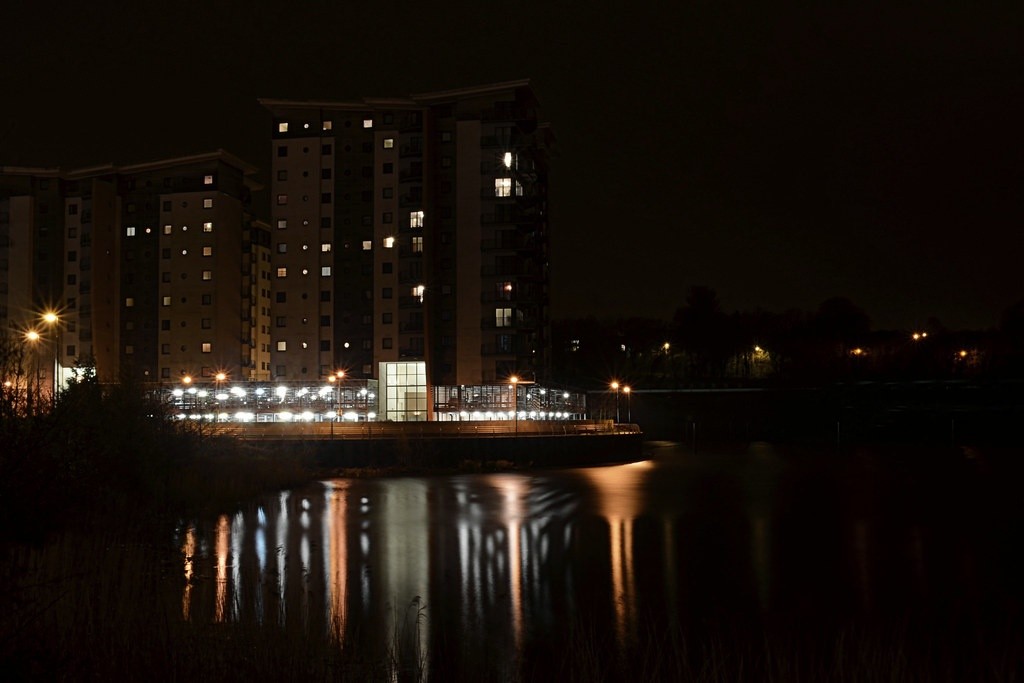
[28,330,40,413]
[610,381,621,424]
[511,377,519,438]
[328,376,336,440]
[216,373,225,394]
[338,372,345,422]
[183,377,191,413]
[44,312,60,406]
[623,386,631,423]
